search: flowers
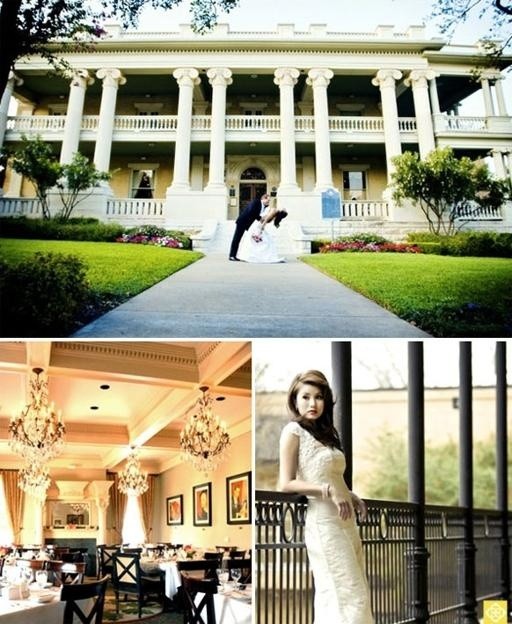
[253,233,264,243]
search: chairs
[1,542,253,623]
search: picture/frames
[165,494,184,525]
[192,482,212,528]
[226,471,251,524]
[67,514,84,525]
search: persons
[236,206,289,264]
[277,370,374,622]
[229,192,270,261]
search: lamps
[4,368,66,500]
[115,447,150,499]
[178,384,231,461]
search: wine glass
[216,568,241,593]
[36,570,48,592]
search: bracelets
[320,482,330,503]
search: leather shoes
[229,256,240,262]
